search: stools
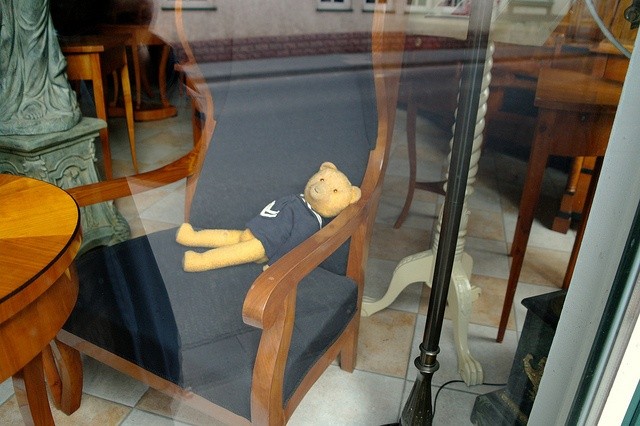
[100,25,177,122]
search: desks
[61,32,138,181]
[1,173,83,426]
[497,67,623,342]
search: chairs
[50,0,410,426]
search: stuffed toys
[175,161,362,271]
